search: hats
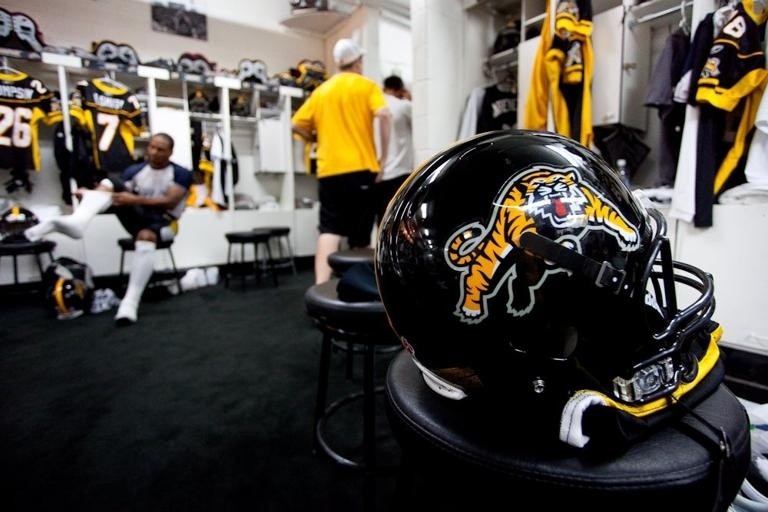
[334,38,369,69]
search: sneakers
[181,267,220,295]
[88,286,116,315]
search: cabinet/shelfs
[461,2,768,360]
[1,45,319,288]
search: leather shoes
[113,295,140,324]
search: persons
[48,131,193,327]
[289,37,393,323]
[367,72,414,239]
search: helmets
[189,88,212,112]
[0,207,41,245]
[39,256,96,320]
[230,93,253,117]
[373,129,717,406]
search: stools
[309,279,399,473]
[327,248,373,278]
[224,227,296,294]
[0,241,59,287]
[118,237,184,297]
[388,346,751,512]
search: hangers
[490,60,517,92]
[677,2,691,39]
